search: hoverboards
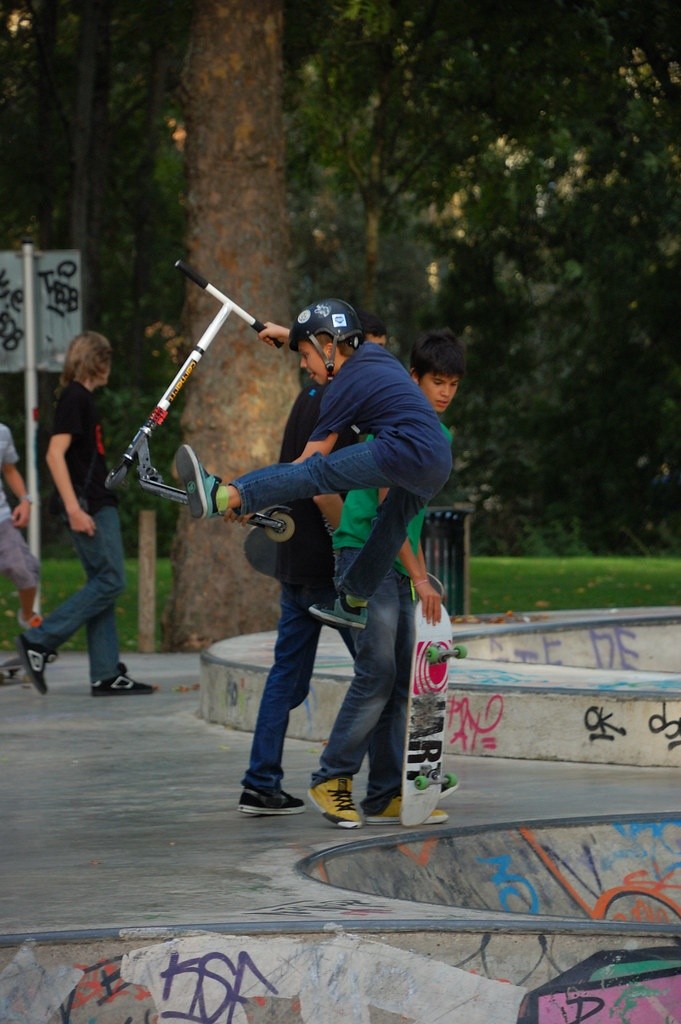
[105,259,295,542]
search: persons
[232,310,457,815]
[0,422,44,628]
[176,295,455,629]
[14,331,155,696]
[311,333,469,827]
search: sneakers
[91,659,153,694]
[175,444,223,519]
[238,779,305,814]
[439,781,460,798]
[308,592,368,629]
[14,633,58,694]
[308,778,362,829]
[364,794,448,823]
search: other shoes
[17,610,45,637]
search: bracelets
[19,494,33,503]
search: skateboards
[399,598,458,830]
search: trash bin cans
[419,504,476,615]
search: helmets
[289,297,363,342]
